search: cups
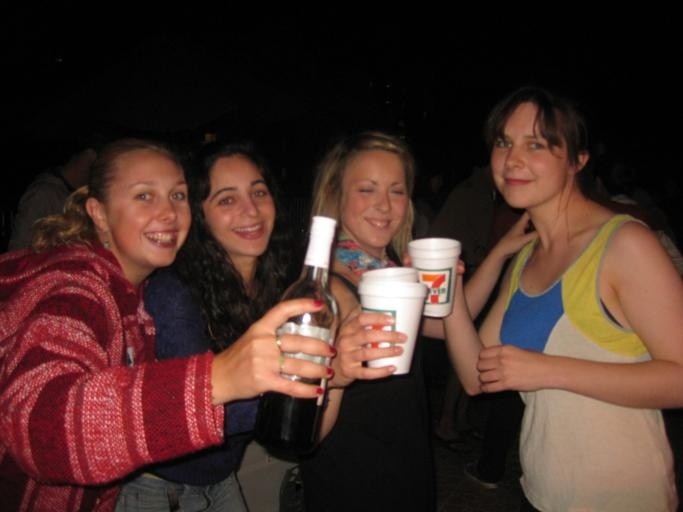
[407,237,462,318]
[355,279,429,376]
[360,266,419,284]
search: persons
[0,134,336,512]
[115,143,409,511]
[311,132,540,512]
[440,80,682,512]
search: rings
[279,356,284,373]
[275,335,284,354]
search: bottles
[254,215,341,461]
